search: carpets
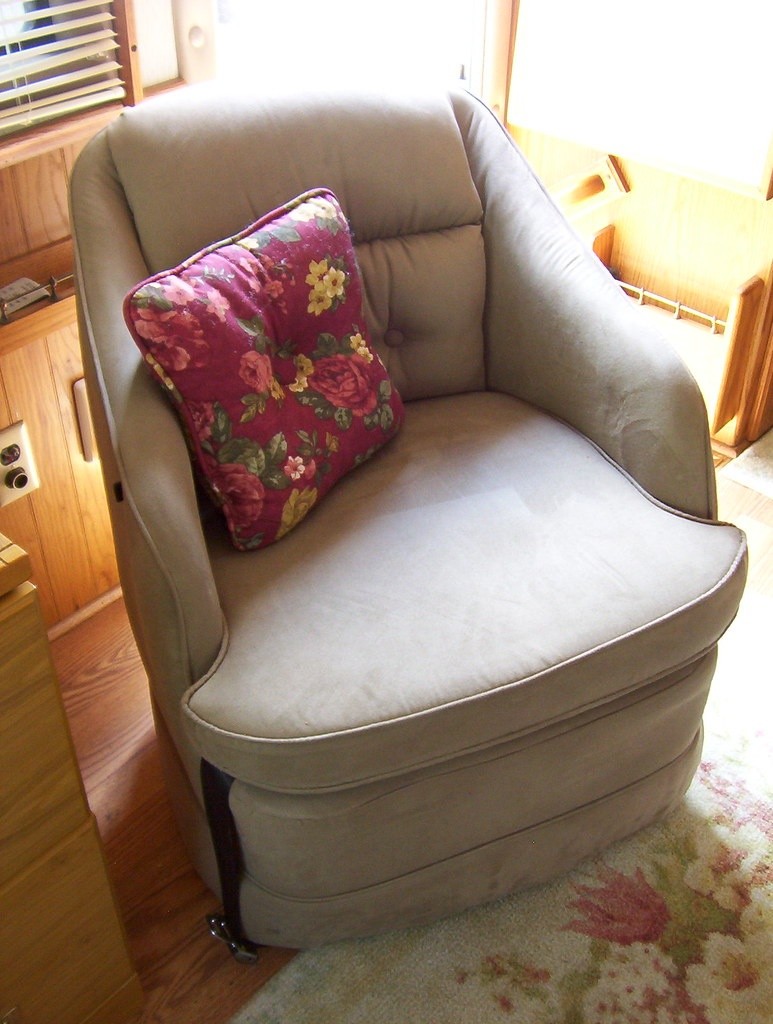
[200,437,773,1024]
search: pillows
[122,189,403,550]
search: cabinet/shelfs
[70,87,748,949]
[0,536,148,1024]
[0,0,142,640]
